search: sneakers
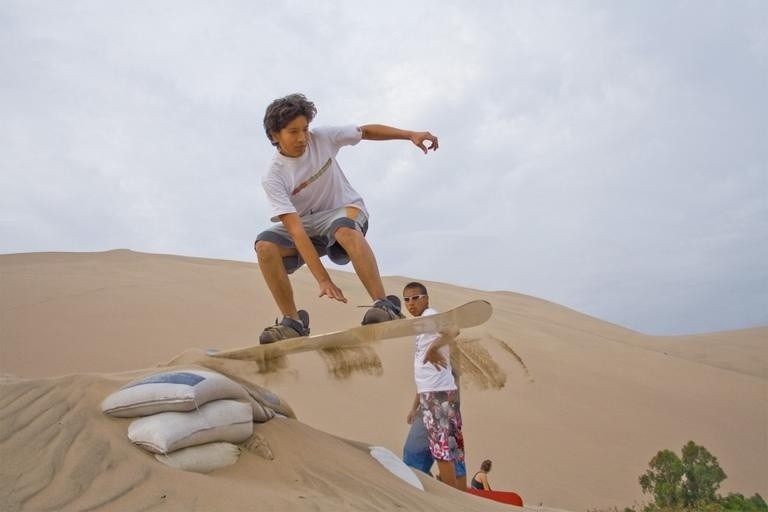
[362,295,400,325]
[260,309,309,344]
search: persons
[253,92,439,344]
[403,281,467,492]
[470,458,492,491]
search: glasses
[404,295,427,301]
